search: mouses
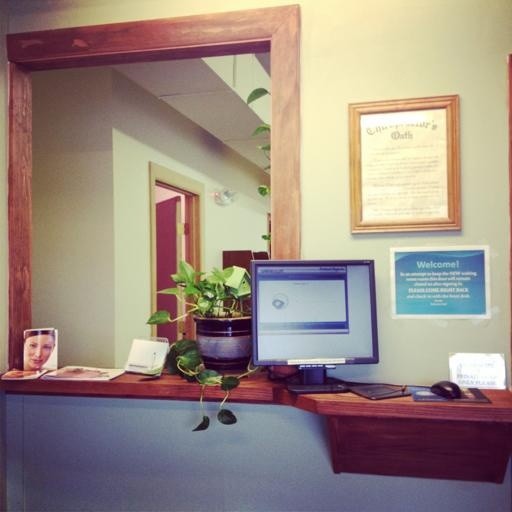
[431,379,461,399]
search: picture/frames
[349,95,461,235]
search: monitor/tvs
[248,257,379,395]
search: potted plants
[147,260,262,432]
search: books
[43,364,127,381]
[1,367,49,379]
[23,328,58,370]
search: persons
[24,331,56,371]
